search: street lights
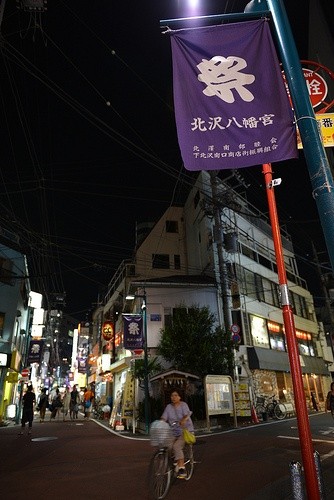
[126,293,150,436]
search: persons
[160,391,192,479]
[19,386,94,433]
[324,382,334,417]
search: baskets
[149,427,175,447]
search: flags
[28,342,43,363]
[167,18,298,171]
[122,314,143,350]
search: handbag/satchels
[184,429,197,445]
[85,400,91,408]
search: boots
[21,428,25,434]
[28,427,32,433]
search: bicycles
[254,394,287,420]
[148,442,195,500]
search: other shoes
[178,468,187,477]
